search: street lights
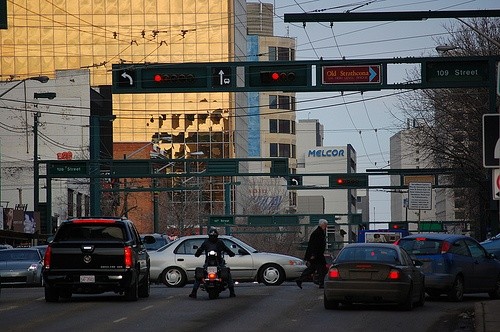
[436,42,500,234]
[32,91,57,212]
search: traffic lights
[393,224,404,229]
[152,73,193,84]
[261,71,295,81]
[338,179,359,184]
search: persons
[188,228,237,299]
[295,219,327,290]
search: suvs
[44,215,151,300]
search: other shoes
[319,284,324,288]
[296,279,303,289]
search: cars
[477,233,500,262]
[323,243,425,311]
[146,234,309,286]
[395,233,500,300]
[138,232,174,257]
[0,247,43,285]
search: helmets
[208,229,219,240]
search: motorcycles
[192,245,229,300]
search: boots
[188,288,198,299]
[228,286,237,298]
